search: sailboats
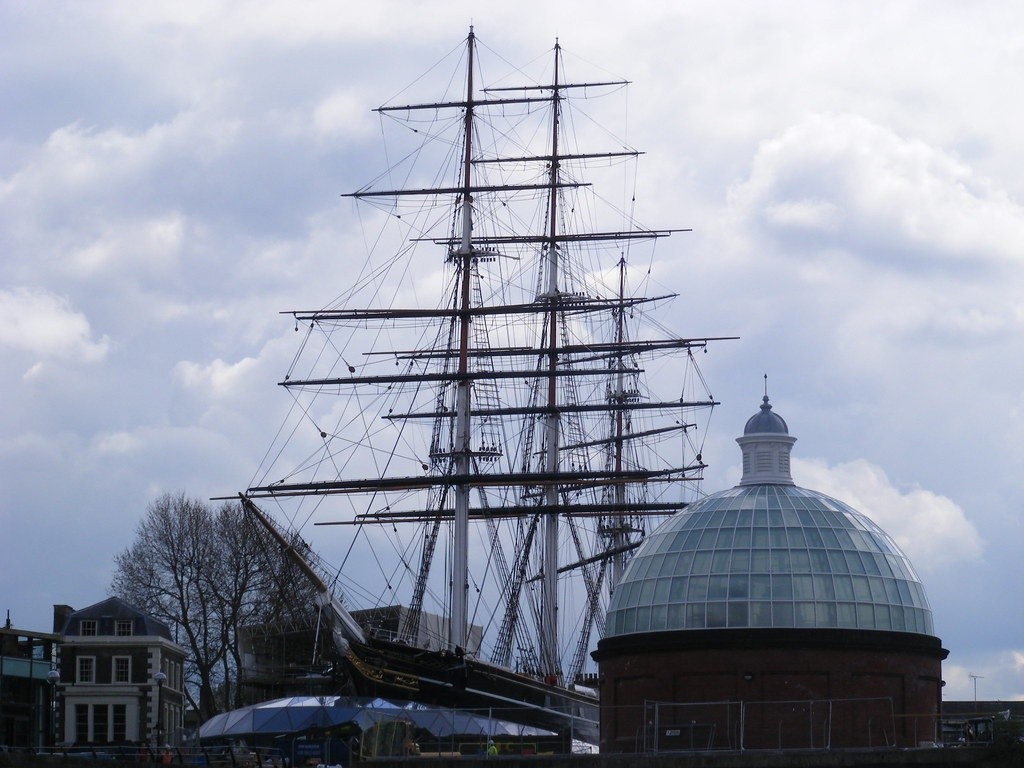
[209,23,739,747]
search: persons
[483,740,498,756]
[232,741,247,754]
[544,670,557,685]
[160,744,175,768]
[138,742,155,768]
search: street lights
[47,671,60,754]
[153,672,166,757]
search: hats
[488,740,494,744]
